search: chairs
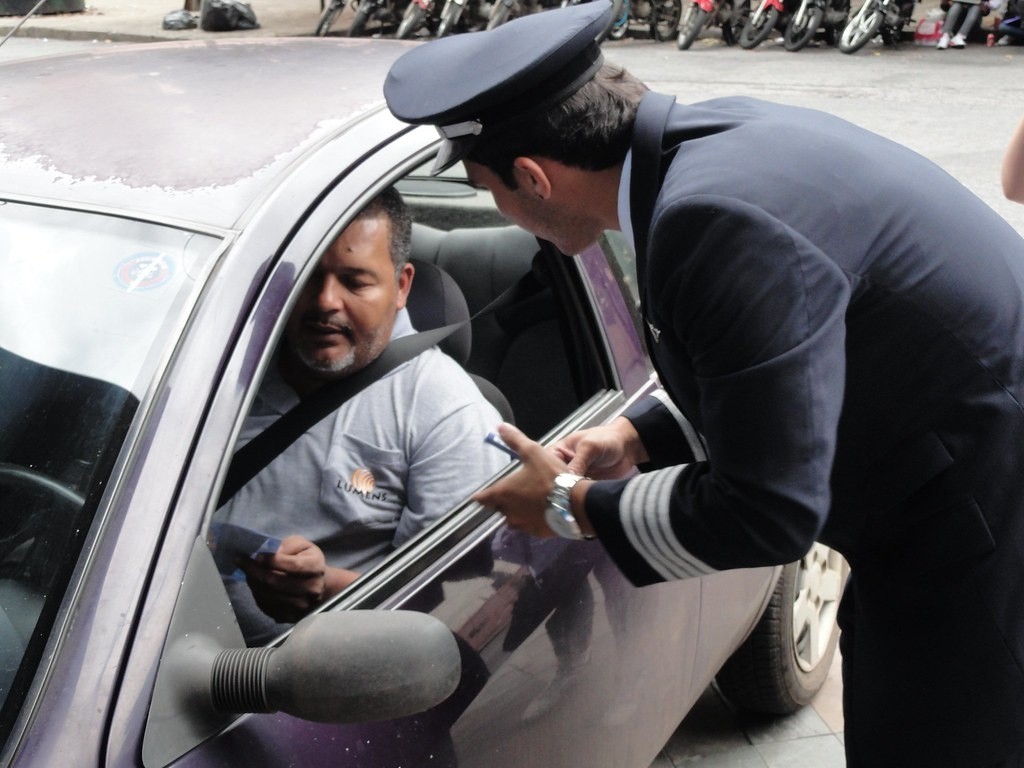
[403,219,582,448]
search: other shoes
[935,35,950,50]
[951,35,967,48]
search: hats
[382,1,614,178]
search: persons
[936,1,1024,51]
[383,0,1024,768]
[205,182,511,645]
[503,523,641,722]
[1002,117,1024,205]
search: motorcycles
[312,0,916,54]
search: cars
[1,34,840,768]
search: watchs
[544,472,595,541]
